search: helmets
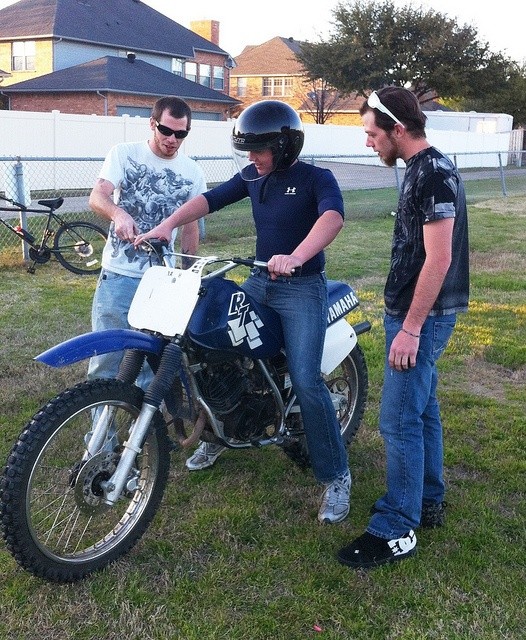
[231,101,305,182]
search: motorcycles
[0,234,371,582]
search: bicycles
[1,195,108,274]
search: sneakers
[168,438,175,454]
[370,501,448,529]
[319,468,353,524]
[337,530,418,569]
[69,444,121,489]
[186,437,236,471]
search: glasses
[367,91,405,129]
[155,119,189,139]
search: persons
[133,101,352,525]
[71,96,207,483]
[336,87,470,568]
